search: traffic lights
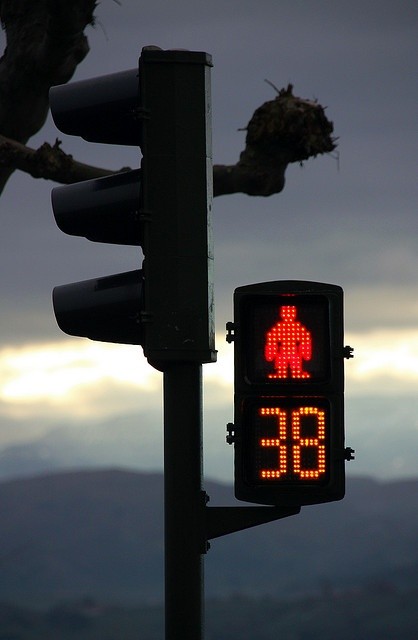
[50,45,212,362]
[225,279,352,503]
